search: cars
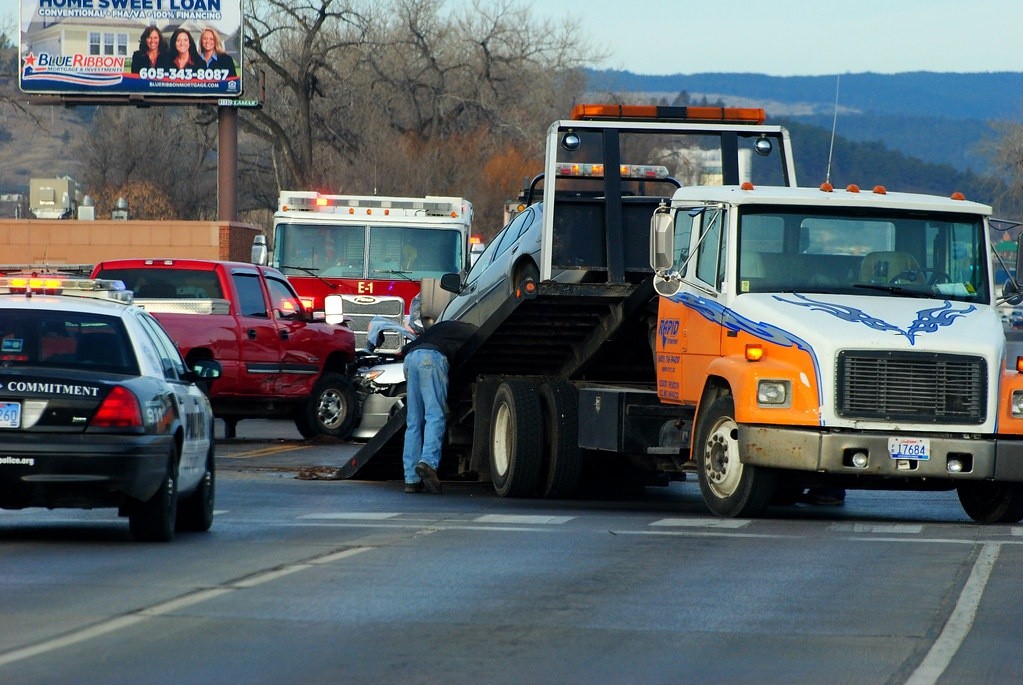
[0,272,223,542]
[356,344,418,440]
[432,201,546,324]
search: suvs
[89,260,357,445]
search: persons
[403,321,480,492]
[158,29,207,78]
[198,28,237,78]
[132,27,169,74]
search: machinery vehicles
[444,105,1023,523]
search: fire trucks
[251,191,475,369]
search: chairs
[76,333,129,367]
[740,251,765,279]
[402,242,440,266]
[11,326,43,360]
[859,251,925,284]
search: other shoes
[415,462,442,494]
[404,482,427,493]
